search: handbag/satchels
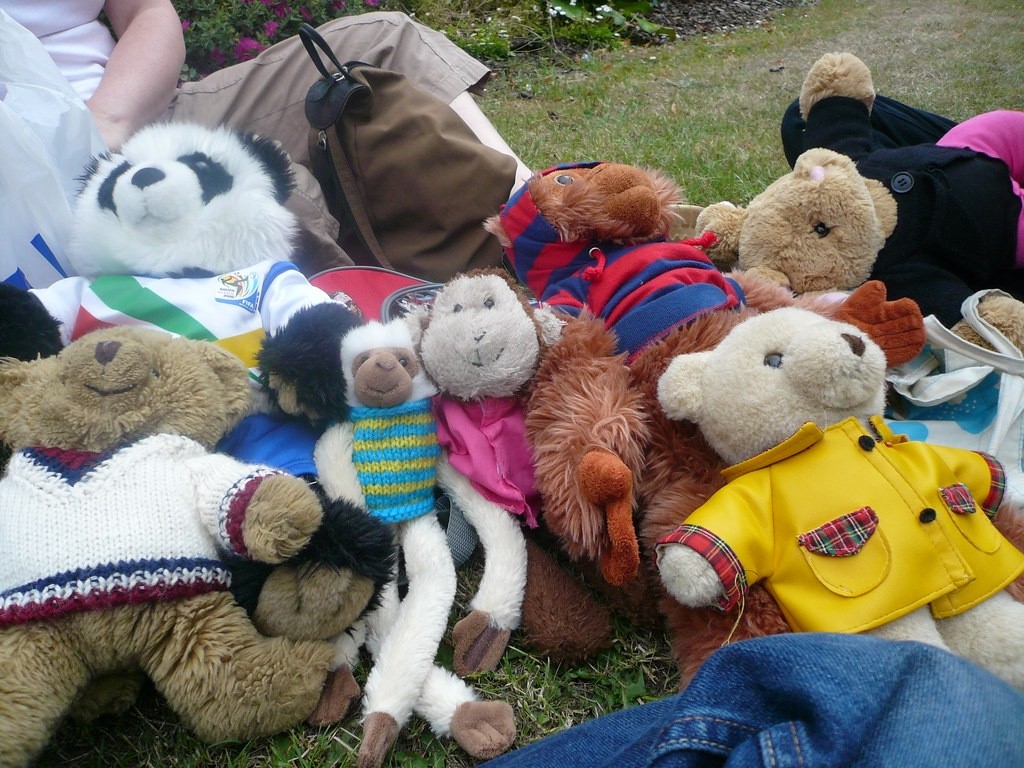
[296,21,517,282]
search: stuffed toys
[0,52,1024,768]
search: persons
[0,0,535,272]
